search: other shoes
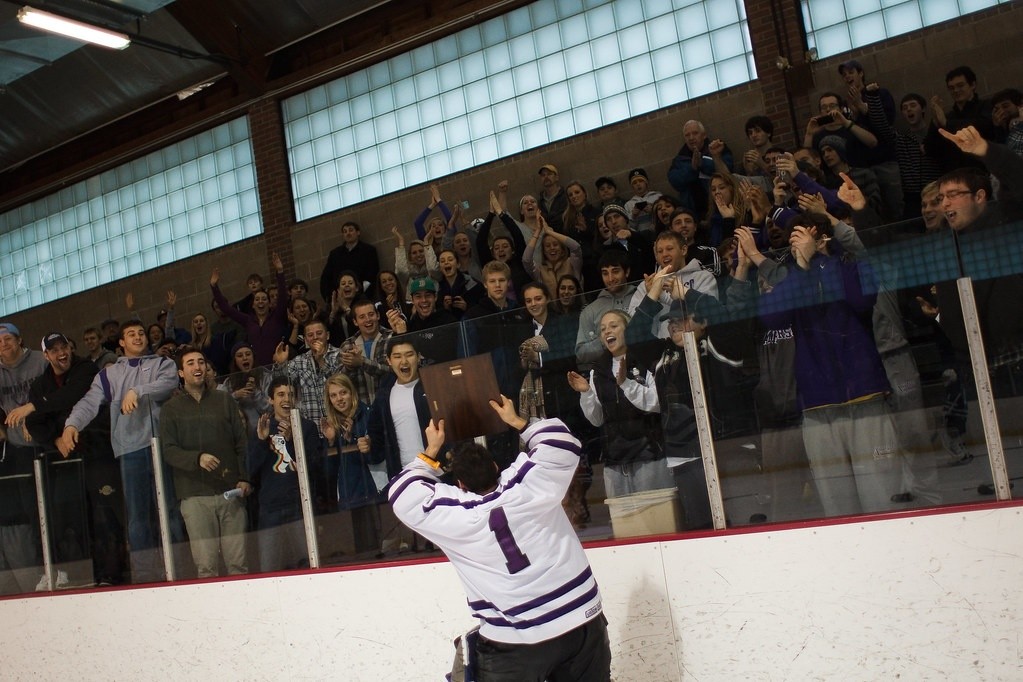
[35,574,56,591]
[55,570,73,589]
[948,442,967,463]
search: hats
[410,277,436,295]
[41,331,69,354]
[629,168,649,189]
[538,164,559,176]
[231,341,256,359]
[595,176,617,188]
[0,322,20,337]
[819,135,848,161]
[659,299,694,322]
[173,326,192,346]
[603,198,630,225]
[101,319,119,330]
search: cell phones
[817,114,834,126]
[635,201,647,210]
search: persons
[1,58,1023,596]
[385,396,613,682]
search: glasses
[936,189,974,201]
[765,157,775,164]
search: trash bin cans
[603,487,679,536]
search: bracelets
[748,251,760,257]
[345,306,351,312]
[846,119,853,130]
[416,453,440,470]
[329,317,335,319]
[399,244,404,246]
[424,244,429,246]
[532,235,538,239]
